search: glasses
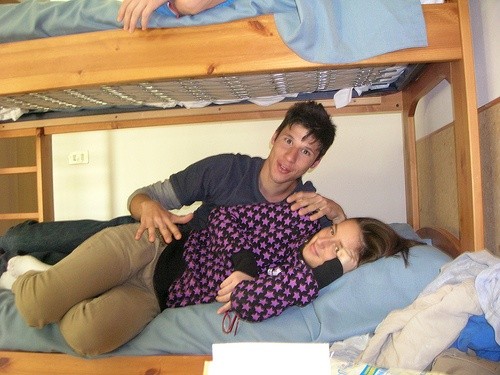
[222,309,241,336]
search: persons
[0,202,426,356]
[1,100,336,275]
[116,0,234,33]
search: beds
[0,0,484,375]
